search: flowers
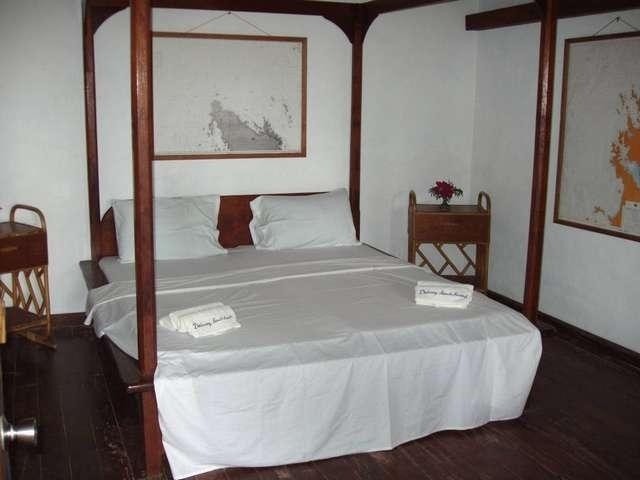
[429,179,463,204]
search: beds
[79,192,540,480]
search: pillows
[112,188,360,263]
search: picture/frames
[149,31,307,161]
[552,32,639,246]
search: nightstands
[0,203,58,351]
[406,191,491,295]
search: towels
[159,302,241,338]
[413,279,475,308]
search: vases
[439,200,450,212]
